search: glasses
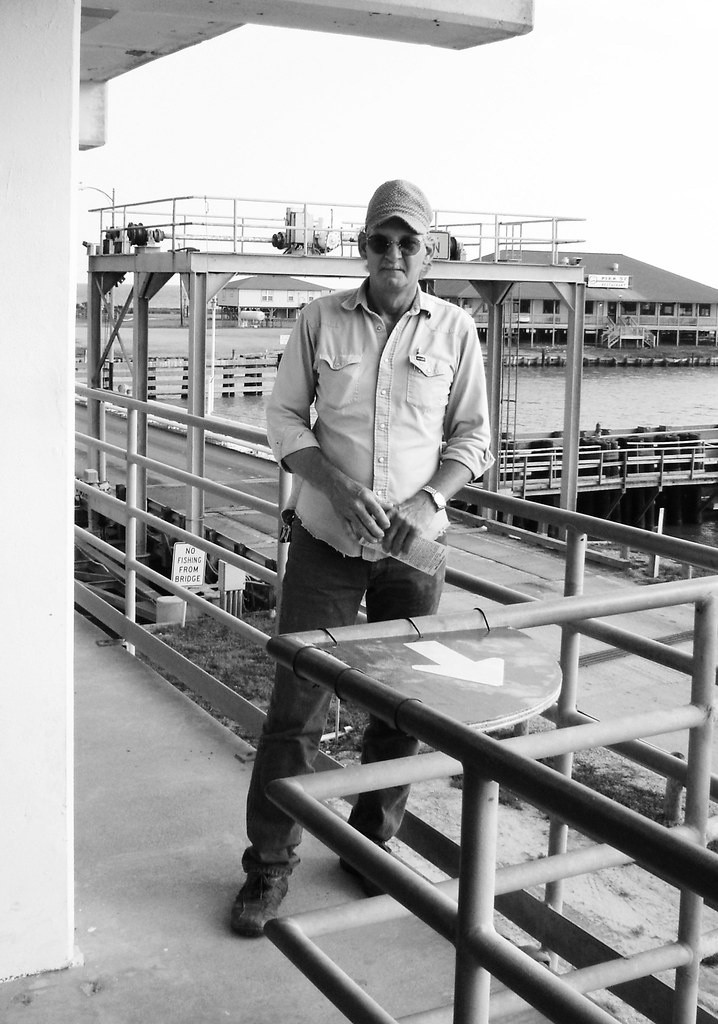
[366,234,421,255]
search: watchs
[420,484,447,512]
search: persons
[229,179,497,936]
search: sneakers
[229,872,288,936]
[340,845,392,897]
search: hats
[365,179,433,234]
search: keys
[279,512,294,542]
